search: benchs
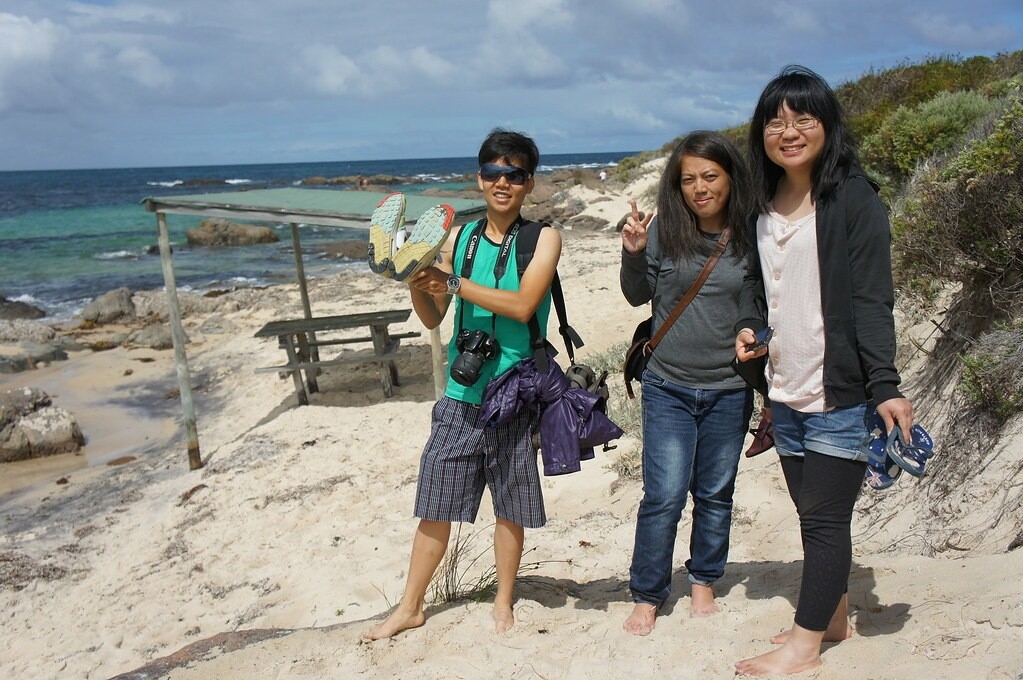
[254,332,422,405]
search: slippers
[863,404,932,490]
[744,408,776,457]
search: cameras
[745,326,775,352]
[450,329,501,387]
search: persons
[361,130,562,644]
[731,62,913,676]
[599,172,607,182]
[620,130,754,635]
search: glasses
[479,163,531,185]
[765,115,820,135]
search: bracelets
[738,328,754,335]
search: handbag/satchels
[623,317,654,398]
[564,363,610,414]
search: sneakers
[367,191,408,277]
[388,202,456,284]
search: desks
[255,308,413,405]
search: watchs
[445,274,461,295]
